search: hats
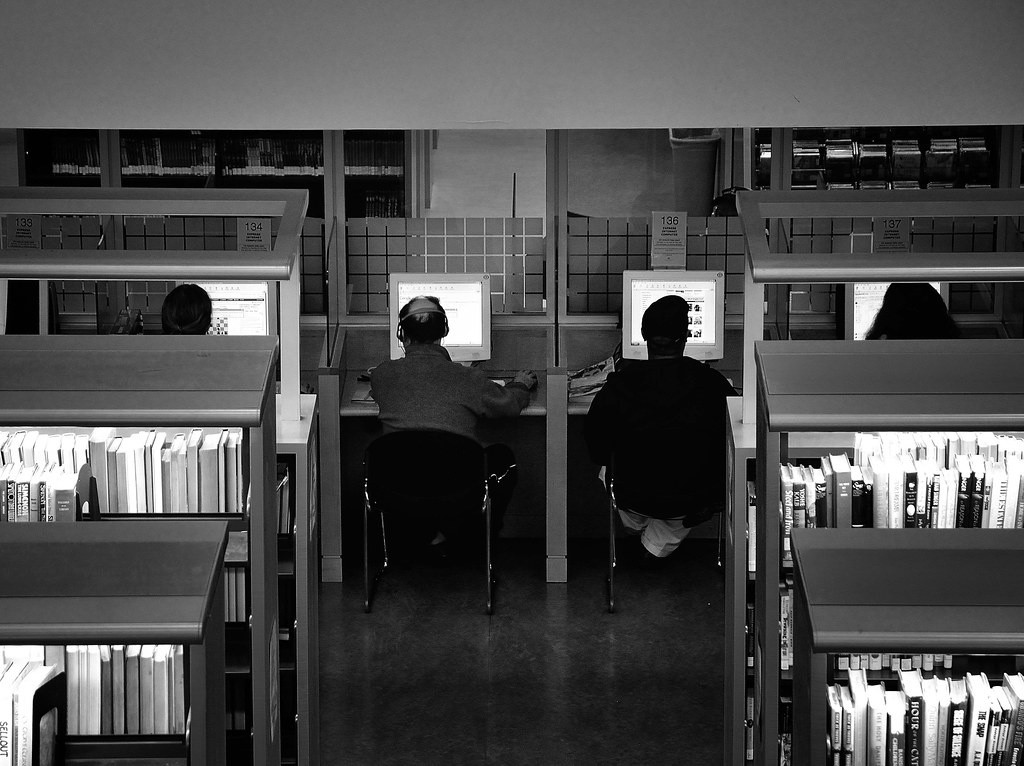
[641,296,690,341]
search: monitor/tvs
[390,272,491,367]
[622,270,724,361]
[175,279,277,335]
[843,283,949,341]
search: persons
[370,297,539,557]
[161,284,212,335]
[582,296,740,563]
[865,283,964,339]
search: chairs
[600,402,726,611]
[360,425,517,618]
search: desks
[341,366,548,418]
[566,369,744,417]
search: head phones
[395,309,449,343]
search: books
[569,358,615,403]
[49,129,406,218]
[1,428,243,522]
[0,567,246,765]
[779,580,1024,765]
[747,482,757,571]
[351,385,375,404]
[747,604,754,666]
[277,468,289,535]
[780,431,1024,562]
[759,136,992,191]
[747,688,753,760]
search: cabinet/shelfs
[722,185,1024,766]
[0,187,316,766]
